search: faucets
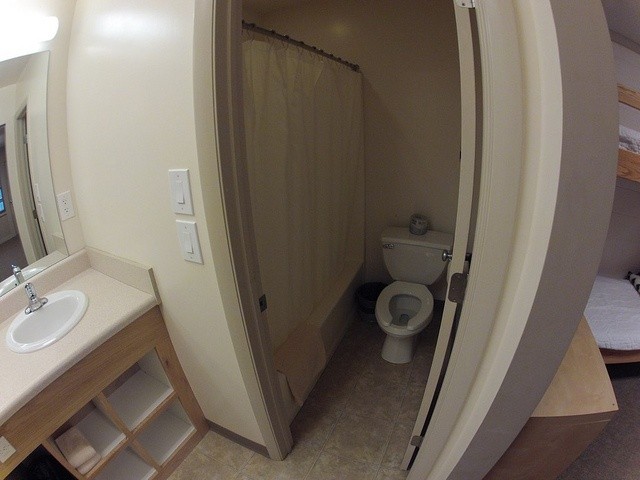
[24,283,48,314]
[11,264,25,284]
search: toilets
[376,225,454,365]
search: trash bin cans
[354,282,388,314]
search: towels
[55,427,95,470]
[77,453,102,476]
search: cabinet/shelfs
[483,314,619,479]
[0,308,210,480]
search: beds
[585,35,638,362]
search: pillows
[626,272,640,295]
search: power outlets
[58,191,75,222]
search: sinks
[7,289,88,354]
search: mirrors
[0,51,70,283]
